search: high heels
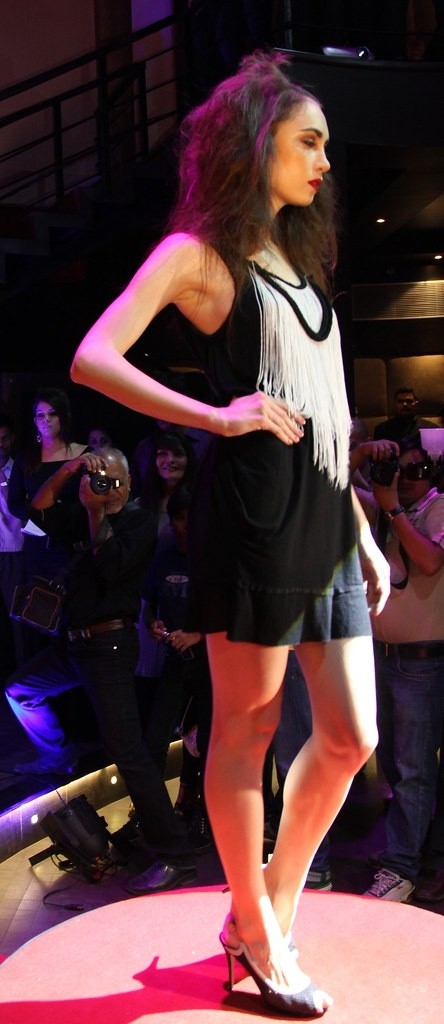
[219,912,332,1017]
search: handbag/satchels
[17,581,66,632]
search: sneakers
[362,867,416,903]
[305,869,333,892]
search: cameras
[81,463,120,496]
[161,632,176,646]
[368,448,399,487]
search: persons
[351,438,444,903]
[70,51,391,1019]
[5,448,198,894]
[0,388,444,892]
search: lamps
[29,794,128,884]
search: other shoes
[109,810,145,844]
[172,783,198,816]
[416,871,444,900]
[264,822,276,843]
[126,859,198,895]
[190,816,212,850]
[14,751,94,776]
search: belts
[67,619,133,641]
[373,639,444,659]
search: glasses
[397,399,414,403]
[395,461,431,481]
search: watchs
[383,507,408,522]
